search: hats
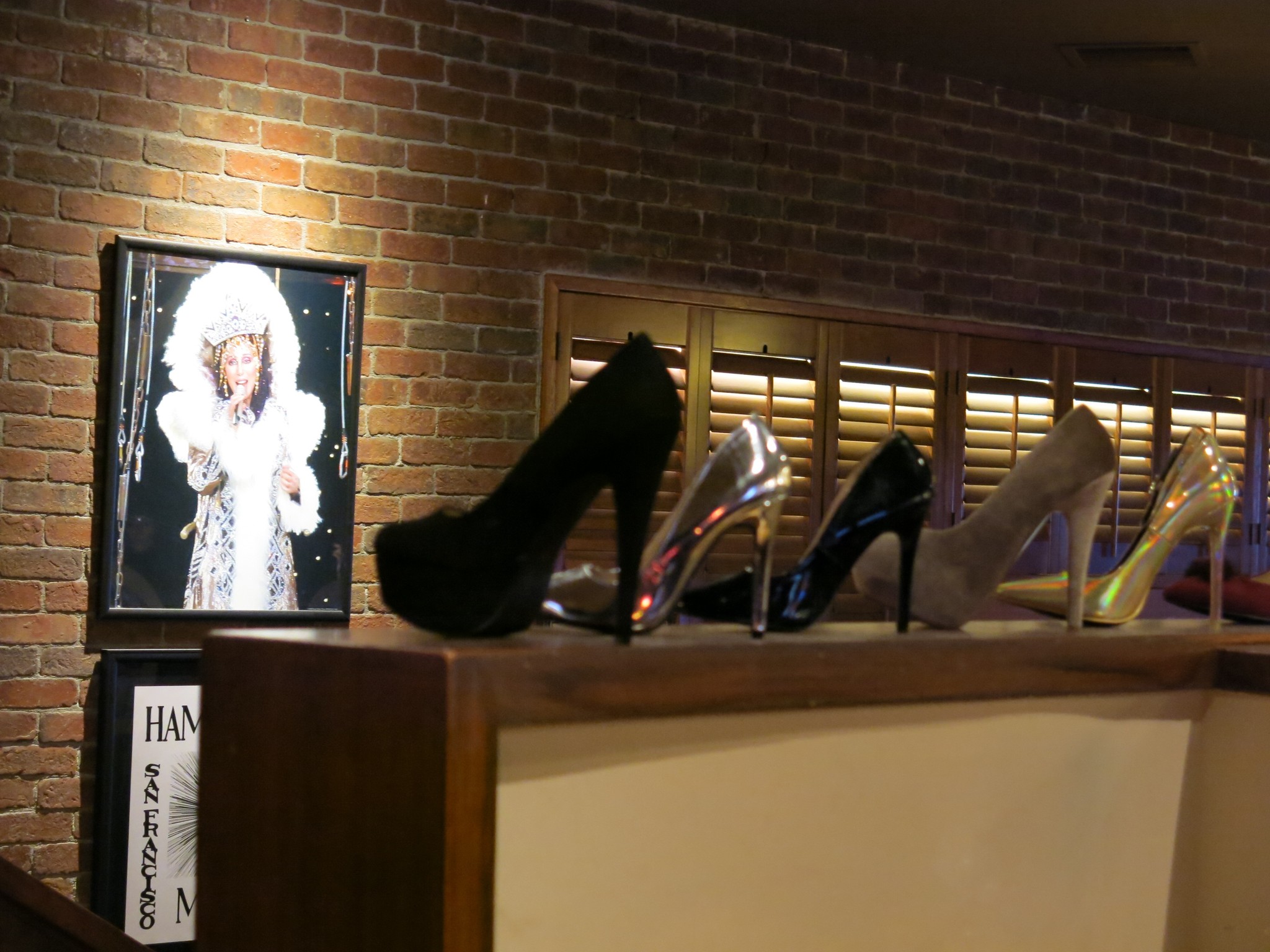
[160,259,300,406]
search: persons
[154,261,327,615]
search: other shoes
[1164,569,1270,622]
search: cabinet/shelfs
[194,606,1270,952]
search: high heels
[994,426,1239,632]
[675,427,936,634]
[542,410,792,640]
[373,331,683,645]
[852,404,1118,633]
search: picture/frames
[88,643,201,952]
[91,232,371,630]
